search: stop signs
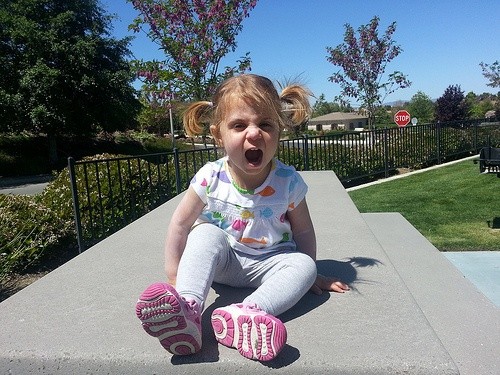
[393,109,411,128]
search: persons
[135,74,350,362]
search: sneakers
[211,302,287,362]
[136,282,203,356]
